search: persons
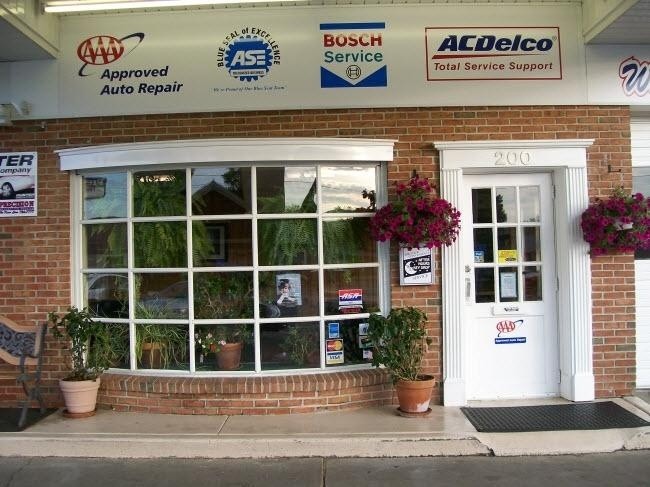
[0,182,15,199]
[277,279,297,307]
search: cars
[87,273,303,326]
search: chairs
[0,315,47,427]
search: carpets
[459,400,650,431]
[1,405,59,431]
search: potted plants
[47,298,249,414]
[363,306,437,412]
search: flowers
[365,171,459,248]
[580,190,650,256]
